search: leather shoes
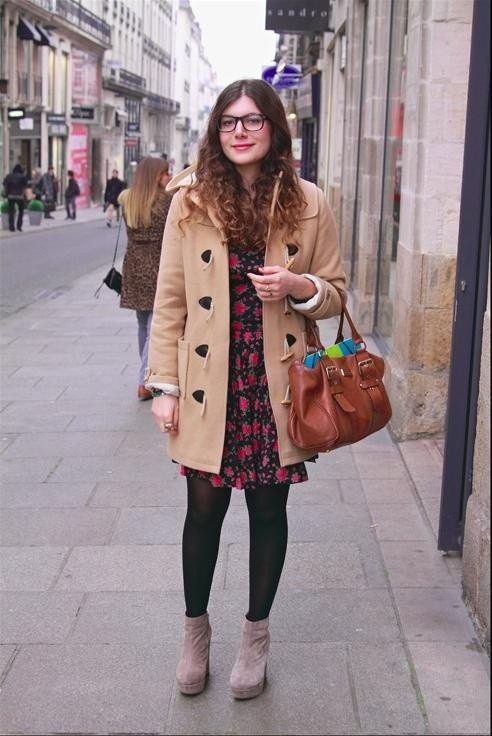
[137,385,153,402]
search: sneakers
[9,224,23,232]
[106,220,121,227]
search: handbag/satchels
[104,268,123,294]
[1,185,10,197]
[288,284,393,451]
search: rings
[266,285,270,292]
[164,424,172,428]
[269,292,272,297]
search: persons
[120,156,176,399]
[147,79,348,701]
[3,164,80,231]
[102,170,123,228]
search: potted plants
[1,199,45,230]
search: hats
[216,113,270,132]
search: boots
[176,611,212,695]
[230,612,270,700]
[65,210,76,220]
[44,211,54,219]
